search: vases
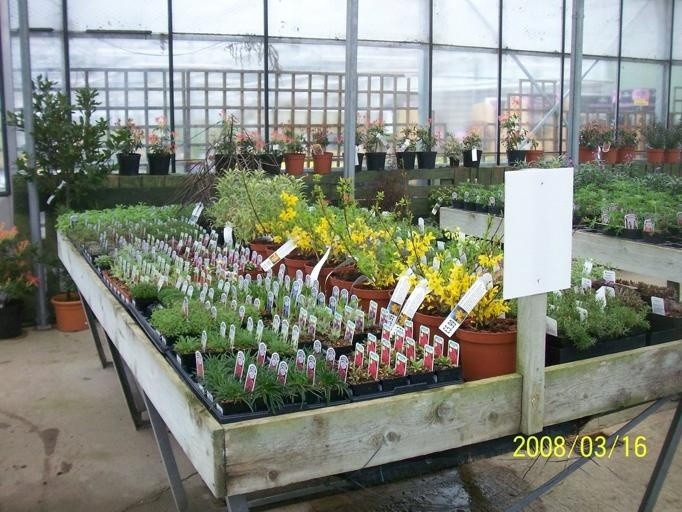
[247,237,518,381]
[115,153,140,175]
[145,152,172,174]
[0,297,22,340]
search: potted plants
[210,112,435,176]
[430,114,681,250]
[52,202,460,417]
[6,72,111,334]
[426,220,681,362]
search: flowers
[146,116,177,154]
[240,167,509,325]
[0,219,39,301]
[108,116,143,155]
